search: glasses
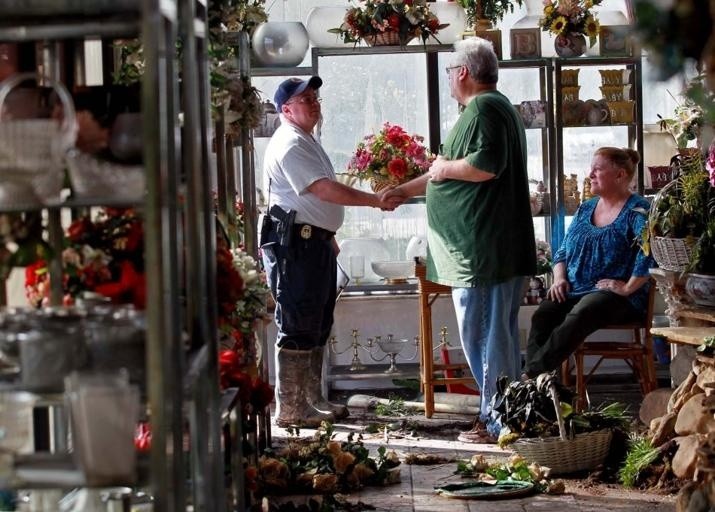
[445,65,461,74]
[284,96,323,103]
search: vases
[558,68,634,125]
[368,176,414,195]
[365,32,414,46]
[555,29,586,57]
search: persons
[522,147,653,380]
[380,35,538,445]
[259,75,402,428]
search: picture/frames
[599,25,633,57]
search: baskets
[650,174,701,272]
[505,375,618,474]
[0,74,80,210]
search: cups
[588,108,607,123]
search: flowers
[537,0,603,48]
[326,0,450,53]
[22,183,280,415]
[336,122,438,188]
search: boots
[304,346,351,418]
[274,343,336,428]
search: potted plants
[675,149,714,307]
[459,0,522,59]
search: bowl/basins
[371,261,415,278]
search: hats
[274,76,323,111]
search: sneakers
[457,416,497,444]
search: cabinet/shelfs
[312,44,646,391]
[0,0,287,511]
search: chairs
[562,277,659,414]
